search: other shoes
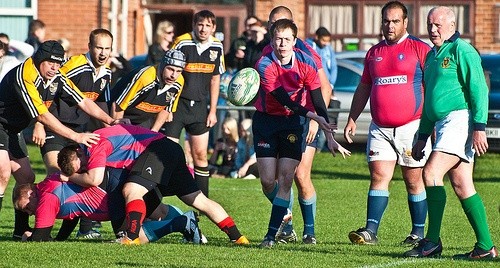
[76,230,101,240]
[111,232,140,246]
[260,236,277,249]
[301,235,317,245]
[13,227,34,241]
[229,236,249,245]
[275,229,298,244]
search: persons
[115,50,187,132]
[343,0,433,245]
[12,172,204,245]
[146,22,175,65]
[260,6,332,246]
[164,10,226,216]
[307,26,338,153]
[402,6,496,261]
[24,19,45,51]
[207,15,267,179]
[0,40,132,240]
[57,125,249,245]
[0,33,21,84]
[33,28,114,238]
[253,19,352,250]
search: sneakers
[403,237,443,257]
[349,227,378,245]
[400,234,424,246]
[452,242,496,261]
[182,210,202,245]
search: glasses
[0,40,9,50]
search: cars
[317,50,374,153]
[480,53,500,155]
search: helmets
[160,49,186,78]
[34,40,65,71]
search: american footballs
[228,68,261,106]
